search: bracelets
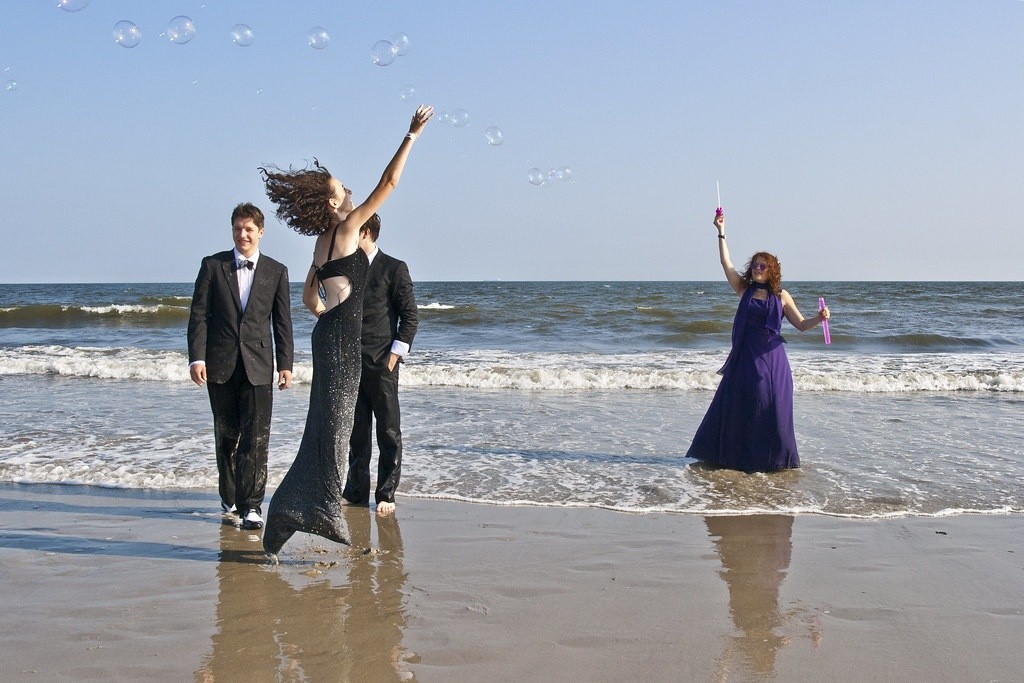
[405,131,418,141]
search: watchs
[718,234,726,240]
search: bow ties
[230,258,254,274]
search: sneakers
[243,509,263,529]
[221,498,239,514]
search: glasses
[750,263,769,270]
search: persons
[341,212,421,515]
[685,212,830,473]
[256,105,435,556]
[187,202,294,529]
